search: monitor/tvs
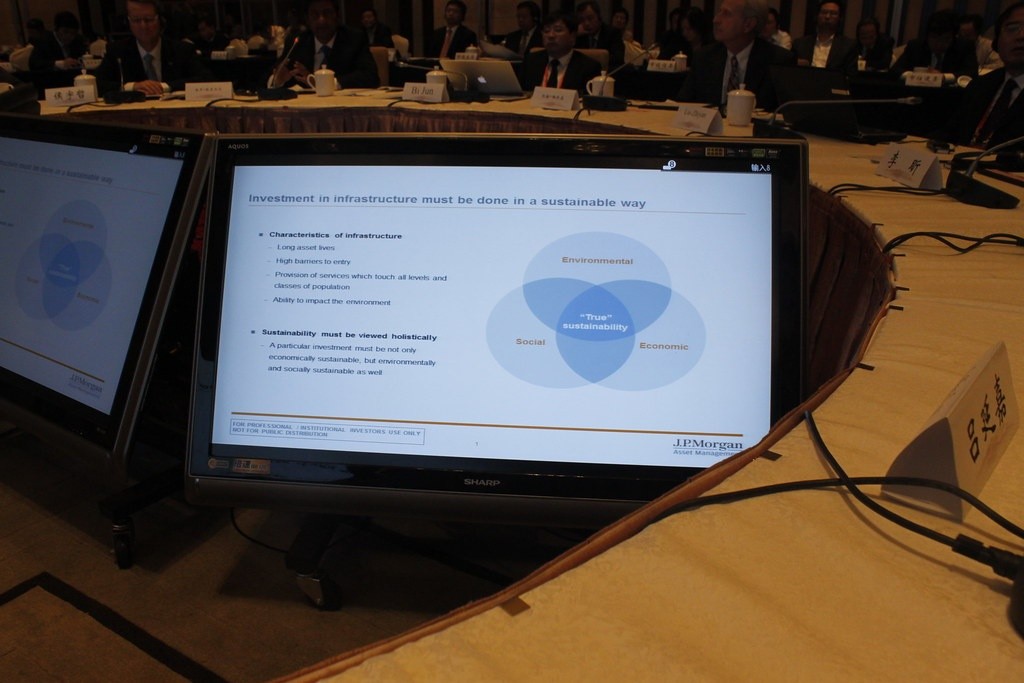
[194,133,815,609]
[1,110,218,570]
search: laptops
[438,60,527,96]
[773,64,908,143]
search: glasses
[999,23,1024,36]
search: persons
[248,0,381,89]
[425,0,479,60]
[690,0,798,118]
[9,0,230,96]
[361,8,394,48]
[765,0,1024,150]
[480,0,713,97]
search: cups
[426,66,448,89]
[82,52,93,60]
[465,44,480,60]
[586,70,615,97]
[673,51,688,72]
[73,68,98,99]
[0,83,9,94]
[226,46,236,60]
[387,48,397,63]
[726,83,757,127]
[306,64,335,97]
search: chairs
[531,49,609,70]
[371,46,389,84]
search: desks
[1,88,1024,683]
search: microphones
[396,61,491,102]
[583,43,658,111]
[753,97,924,140]
[258,36,301,100]
[103,58,146,104]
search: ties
[725,56,740,105]
[975,78,1019,143]
[590,36,596,48]
[520,32,528,50]
[319,45,330,69]
[547,59,561,88]
[440,28,452,56]
[143,53,158,81]
